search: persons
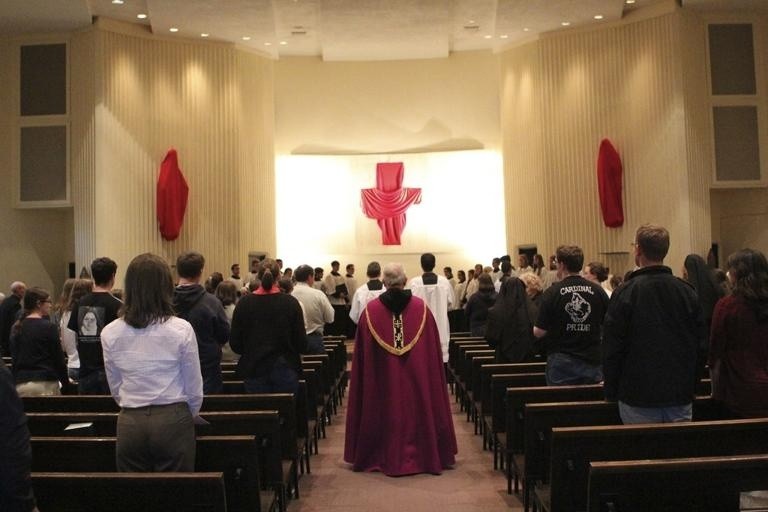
[583,262,624,298]
[443,253,560,365]
[600,222,706,426]
[99,254,208,472]
[0,279,93,398]
[205,260,261,356]
[67,257,124,395]
[170,251,229,396]
[682,254,725,327]
[348,261,388,324]
[533,245,610,387]
[227,258,306,394]
[275,261,357,344]
[406,253,455,364]
[711,248,768,420]
[343,263,459,477]
[1,364,38,512]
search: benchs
[444,332,767,511]
[2,328,351,511]
